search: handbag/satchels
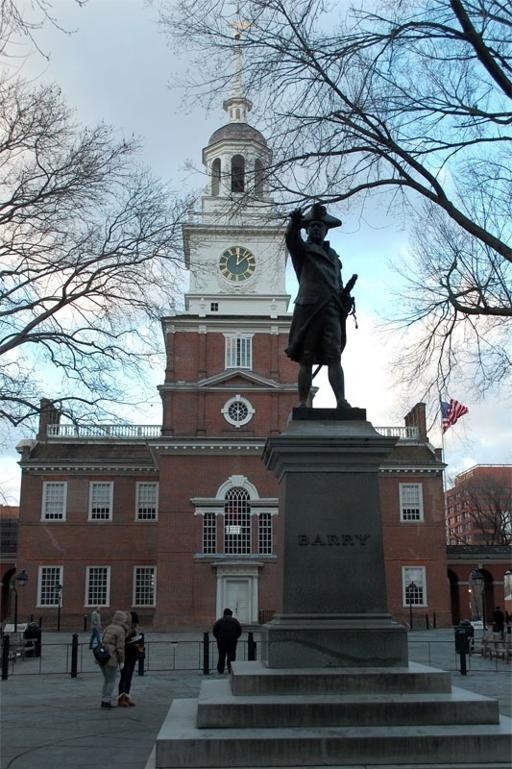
[93,642,113,665]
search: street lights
[55,580,63,632]
[409,581,416,629]
[12,569,26,634]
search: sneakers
[101,701,117,709]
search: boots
[118,692,137,708]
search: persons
[88,605,103,649]
[94,610,133,709]
[492,606,512,632]
[284,202,355,411]
[120,611,146,709]
[212,607,242,676]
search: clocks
[218,247,256,284]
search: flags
[441,399,469,435]
